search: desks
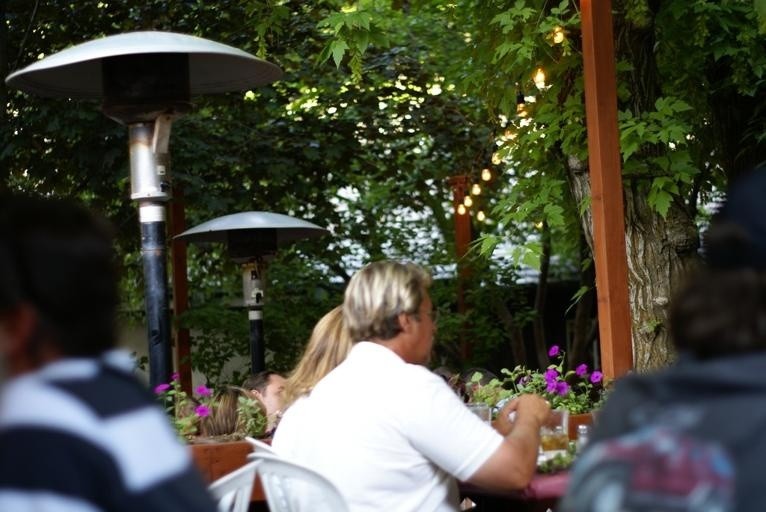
[457,471,570,505]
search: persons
[554,176,766,512]
[201,384,268,439]
[1,193,225,510]
[240,366,286,432]
[271,260,552,511]
[287,303,355,409]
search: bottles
[577,425,590,447]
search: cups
[539,407,571,450]
[464,400,494,427]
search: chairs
[245,451,351,512]
[243,435,273,455]
[206,462,258,512]
